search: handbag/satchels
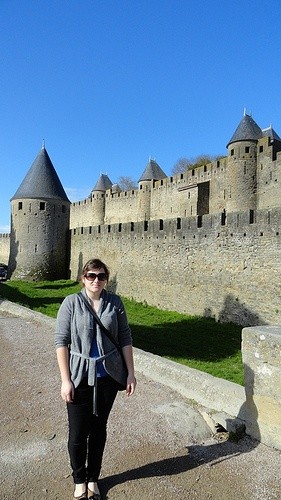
[119,355,128,391]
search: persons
[54,259,137,500]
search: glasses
[83,272,108,281]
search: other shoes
[72,482,87,500]
[87,480,101,500]
